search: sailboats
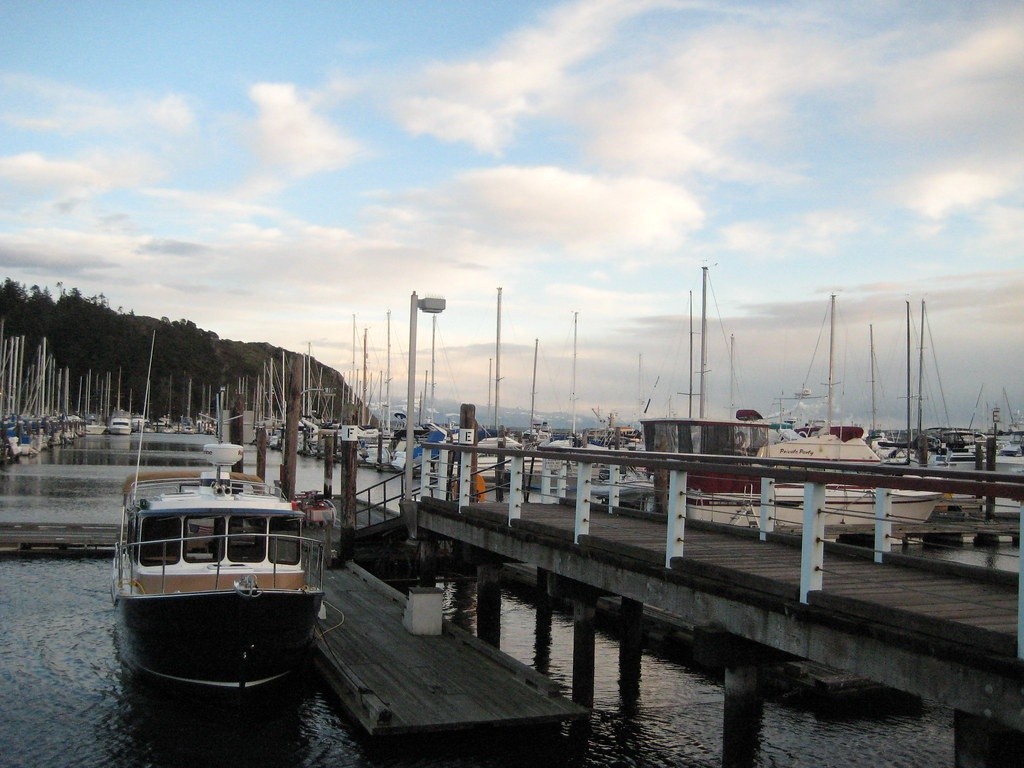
[1,263,1023,539]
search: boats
[106,329,327,718]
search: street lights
[402,290,446,502]
